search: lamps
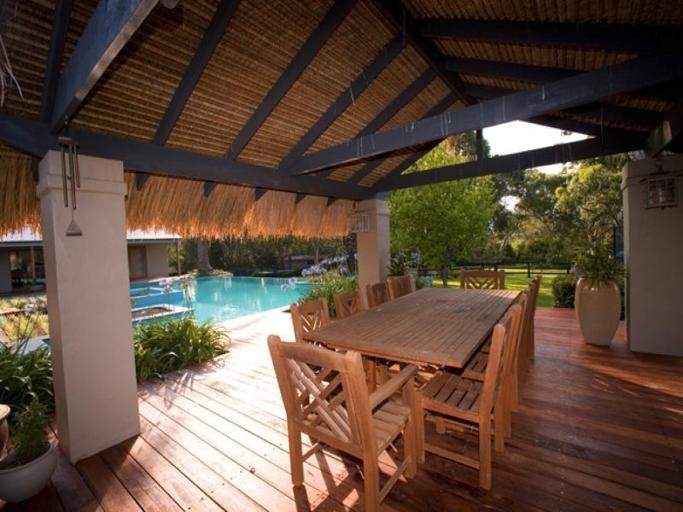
[641,156,678,213]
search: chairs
[387,273,412,298]
[332,287,363,317]
[267,332,424,510]
[458,270,506,290]
[291,297,332,399]
[421,275,543,490]
[366,282,390,306]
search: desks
[303,286,522,464]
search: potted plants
[572,243,633,347]
[0,400,58,502]
